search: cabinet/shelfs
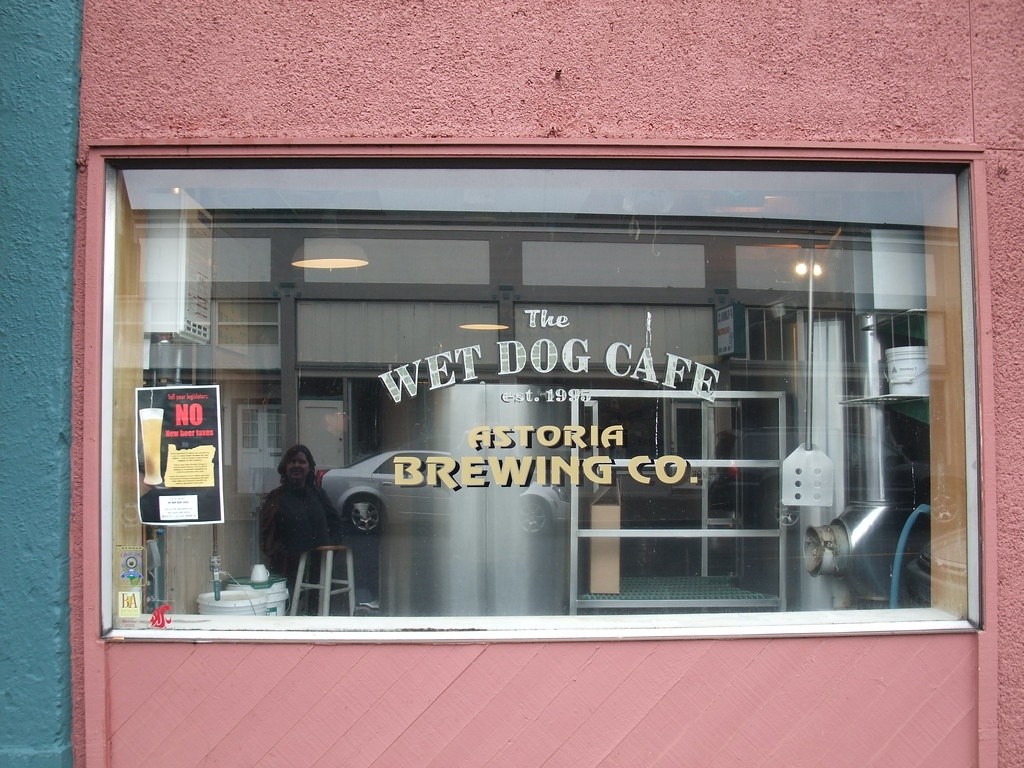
[569,389,787,616]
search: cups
[251,564,270,582]
[139,408,163,485]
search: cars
[312,450,573,540]
[702,424,931,562]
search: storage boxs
[589,475,621,595]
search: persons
[708,431,737,517]
[259,444,341,615]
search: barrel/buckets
[224,576,291,616]
[885,346,928,394]
[197,571,267,615]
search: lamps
[290,238,368,270]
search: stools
[290,545,355,616]
